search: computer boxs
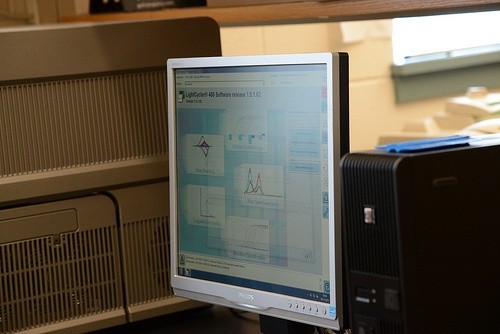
[340,136,500,334]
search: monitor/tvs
[166,52,350,334]
